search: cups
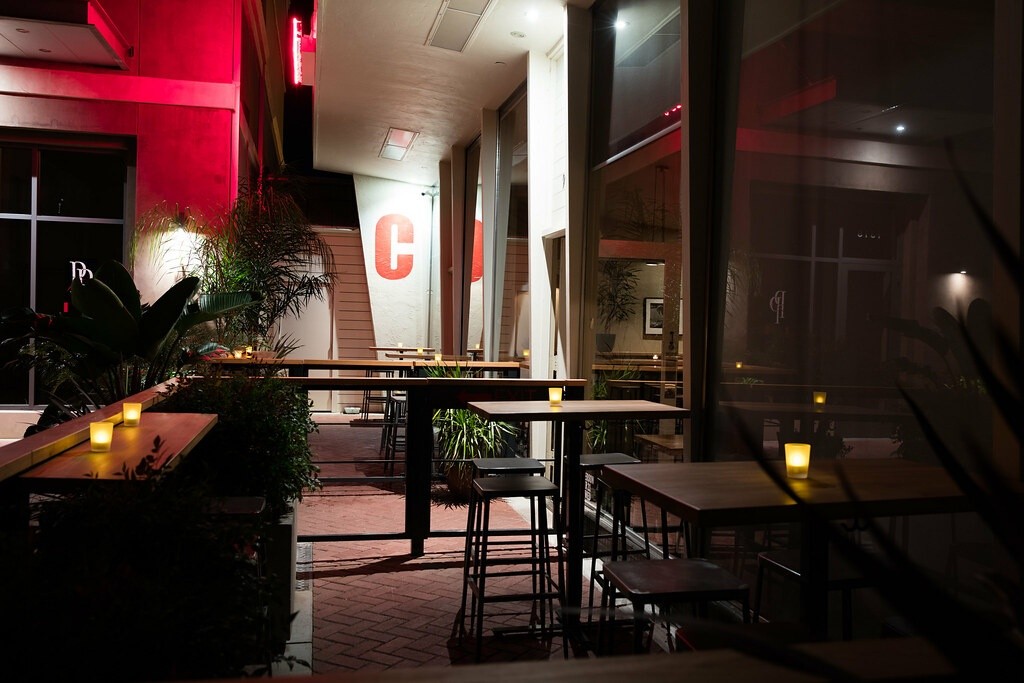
[235,350,242,358]
[123,403,141,426]
[398,342,402,348]
[475,344,479,350]
[435,353,442,363]
[418,347,423,356]
[814,392,826,409]
[90,422,113,453]
[784,443,811,479]
[737,361,743,369]
[524,349,528,357]
[549,388,562,404]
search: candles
[785,443,810,479]
[90,422,113,453]
[124,402,142,426]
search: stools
[349,370,992,666]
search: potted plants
[0,180,344,683]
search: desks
[602,456,993,643]
[467,349,509,362]
[368,346,420,354]
[199,358,520,480]
[19,412,221,493]
[193,375,585,557]
[164,635,968,683]
[593,358,794,458]
[385,353,473,361]
[467,400,692,642]
[720,399,913,582]
[596,351,685,358]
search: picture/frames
[643,297,684,337]
